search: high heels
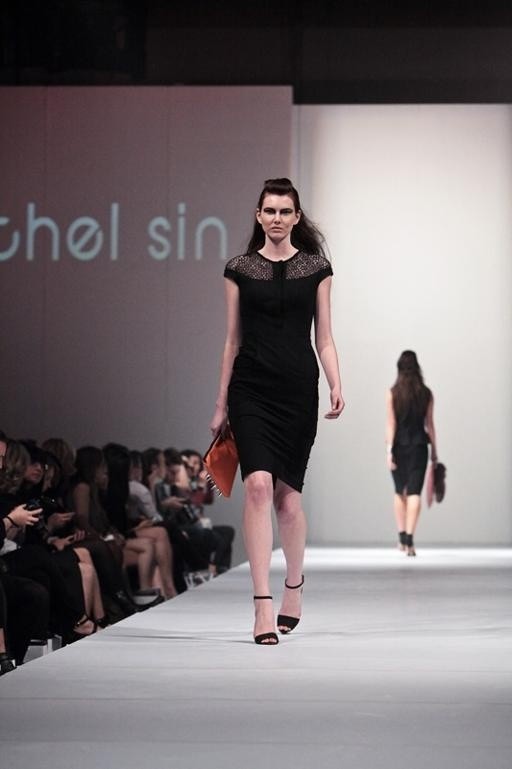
[399,532,416,555]
[1,653,17,670]
[253,596,278,645]
[277,575,305,634]
[73,618,102,635]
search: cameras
[25,497,44,530]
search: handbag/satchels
[203,426,240,499]
[426,463,445,508]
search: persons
[387,351,437,557]
[212,177,345,646]
